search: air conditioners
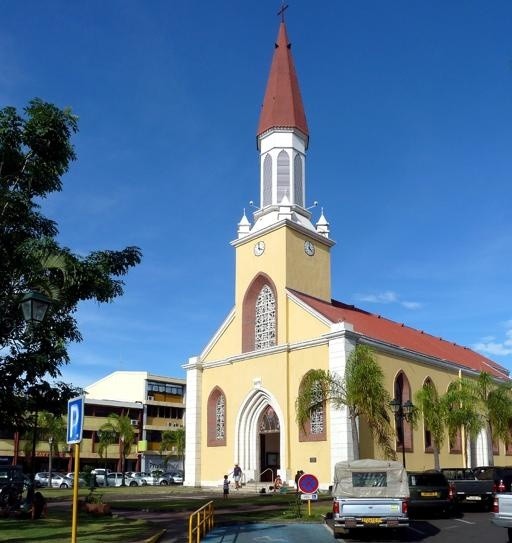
[131,420,138,425]
[173,423,183,428]
[167,422,172,427]
[148,396,154,401]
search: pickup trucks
[329,459,410,539]
[441,466,494,510]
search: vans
[0,464,32,489]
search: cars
[34,468,184,488]
[488,466,512,542]
[407,470,456,514]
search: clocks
[304,240,315,256]
[254,240,265,256]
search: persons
[8,492,48,520]
[274,474,282,489]
[223,474,231,500]
[233,463,242,488]
[295,470,304,492]
